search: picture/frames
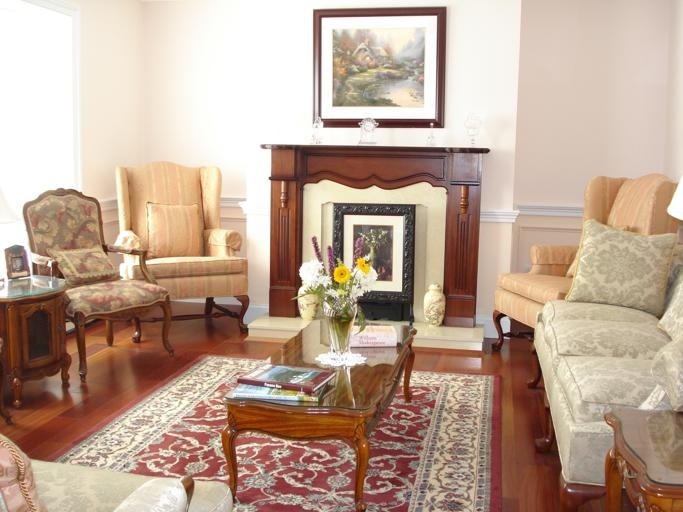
[310,4,447,129]
[330,200,417,304]
[3,243,30,279]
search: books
[229,362,337,404]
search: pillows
[0,433,39,512]
[140,199,208,259]
[562,218,676,316]
[47,242,122,287]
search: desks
[600,403,683,512]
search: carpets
[48,351,502,512]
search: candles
[460,184,469,197]
[280,178,289,196]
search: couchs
[10,447,234,512]
[529,213,683,512]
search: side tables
[0,275,68,408]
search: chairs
[489,175,683,385]
[21,186,175,381]
[112,159,251,342]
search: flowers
[291,234,380,336]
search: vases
[322,300,359,361]
[295,282,318,319]
[422,284,447,327]
